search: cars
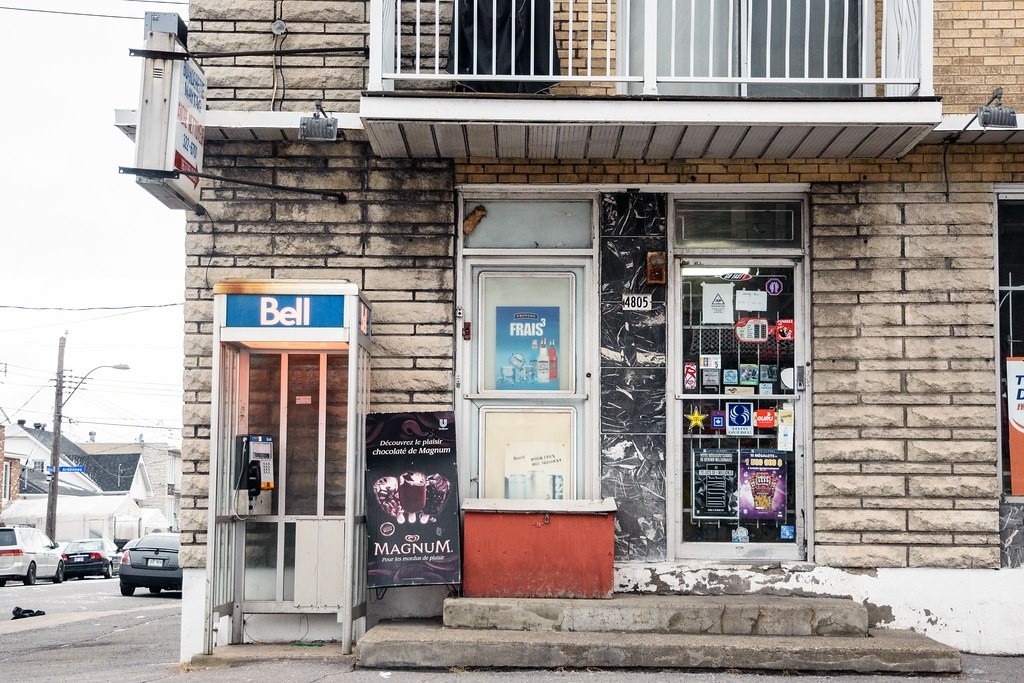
[60,537,122,581]
[119,532,183,596]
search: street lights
[45,363,131,542]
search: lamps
[298,99,347,142]
[951,87,1017,142]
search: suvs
[0,523,65,587]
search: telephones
[234,433,274,496]
